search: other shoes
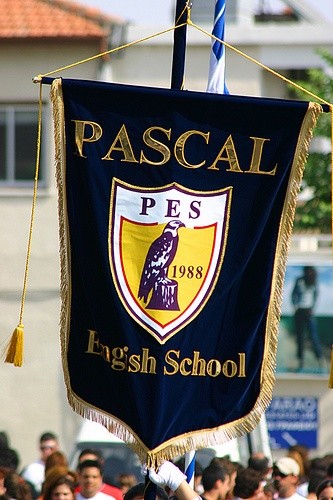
[285,357,326,373]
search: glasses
[39,445,54,451]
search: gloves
[140,458,187,493]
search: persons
[175,457,204,494]
[0,448,38,500]
[288,267,329,373]
[76,460,117,500]
[199,466,230,500]
[288,444,333,500]
[19,432,62,494]
[0,432,10,450]
[123,458,202,500]
[209,453,274,500]
[43,475,77,500]
[37,448,123,500]
[272,457,309,500]
[117,474,137,498]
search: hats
[274,457,300,477]
